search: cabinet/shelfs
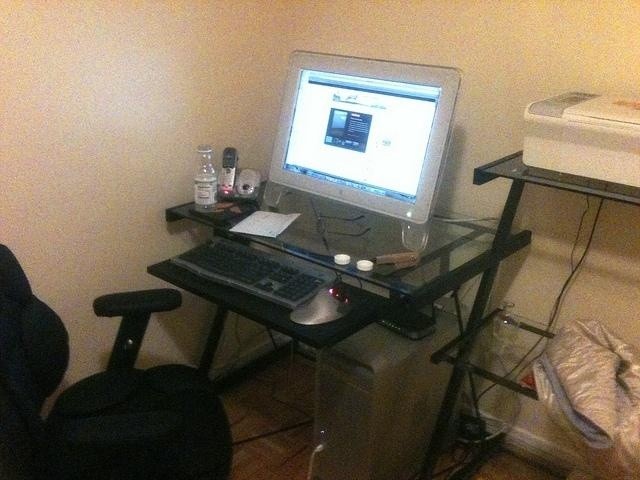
[420,149,639,480]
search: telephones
[216,146,238,202]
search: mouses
[288,286,361,326]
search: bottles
[487,301,524,362]
[193,142,218,211]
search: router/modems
[375,308,438,341]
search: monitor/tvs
[264,49,462,252]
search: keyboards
[172,234,341,309]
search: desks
[146,177,532,480]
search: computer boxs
[311,306,469,480]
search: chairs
[0,243,234,479]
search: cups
[402,208,433,253]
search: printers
[522,90,640,192]
